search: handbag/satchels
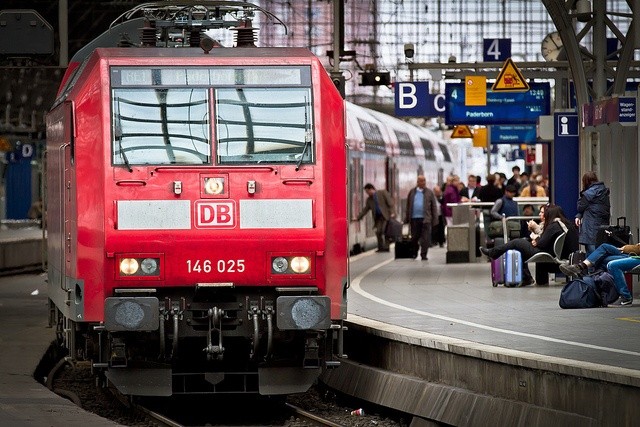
[385,217,402,238]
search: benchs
[525,217,568,288]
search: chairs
[624,263,640,307]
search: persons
[489,183,518,220]
[479,174,503,201]
[526,204,551,239]
[479,205,575,286]
[436,175,462,228]
[458,182,465,191]
[574,171,610,259]
[494,172,504,195]
[351,183,396,252]
[520,171,529,186]
[404,175,439,260]
[520,204,541,237]
[432,185,445,247]
[518,172,546,197]
[459,174,481,202]
[477,175,483,186]
[500,172,508,187]
[507,165,523,196]
[558,242,640,307]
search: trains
[40,0,465,408]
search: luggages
[568,218,586,282]
[395,221,417,258]
[491,212,507,287]
[494,237,510,248]
[505,249,523,287]
[595,216,631,249]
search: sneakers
[560,260,587,277]
[607,296,632,308]
[479,247,498,260]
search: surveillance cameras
[403,43,415,58]
